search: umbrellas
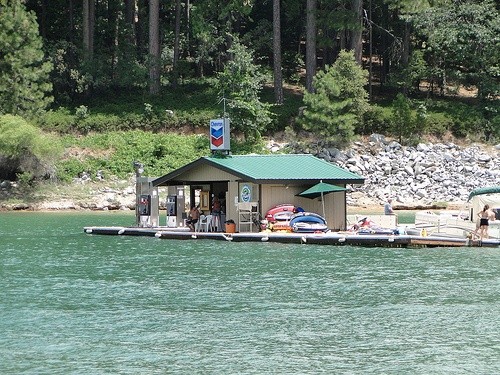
[293,180,349,218]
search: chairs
[184,215,212,232]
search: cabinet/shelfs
[239,202,259,233]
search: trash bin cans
[224,220,238,234]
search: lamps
[285,184,288,190]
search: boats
[407,186,500,245]
[267,203,329,234]
[353,216,396,235]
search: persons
[211,197,226,219]
[477,204,491,240]
[186,204,200,232]
[385,198,400,227]
[472,210,498,240]
